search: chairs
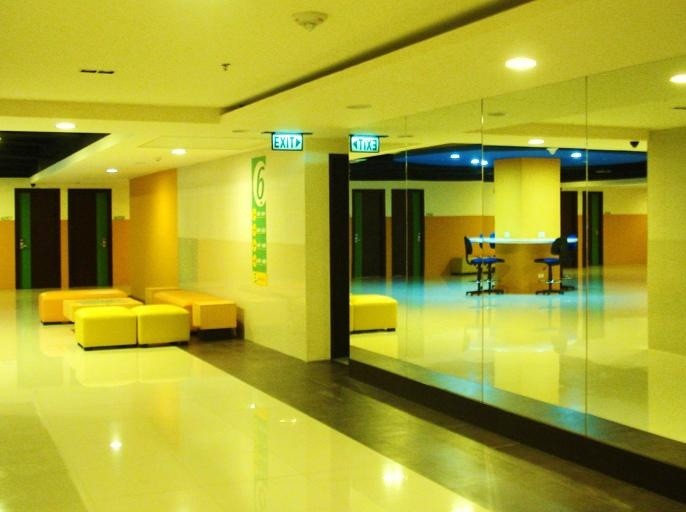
[463,233,574,296]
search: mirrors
[345,55,685,502]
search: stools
[350,292,399,331]
[73,304,191,348]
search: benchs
[38,287,239,337]
